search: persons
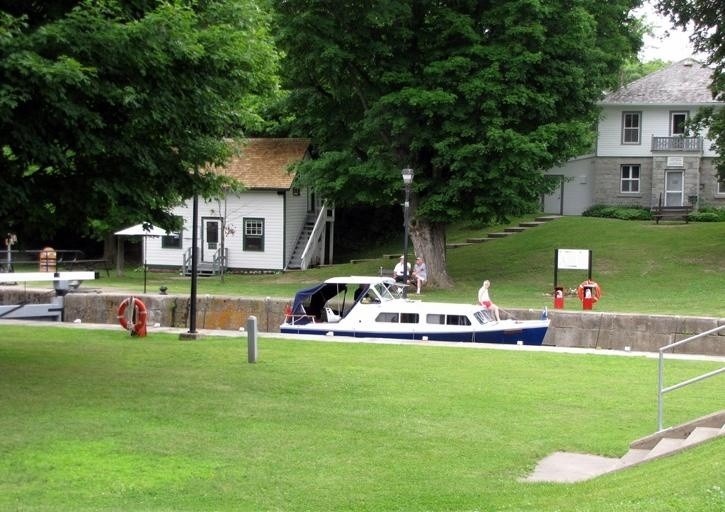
[478,280,501,321]
[354,285,369,302]
[407,257,427,295]
[394,255,411,284]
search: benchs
[652,206,692,224]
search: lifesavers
[577,280,600,303]
[118,298,146,330]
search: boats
[276,275,551,346]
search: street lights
[400,165,416,299]
